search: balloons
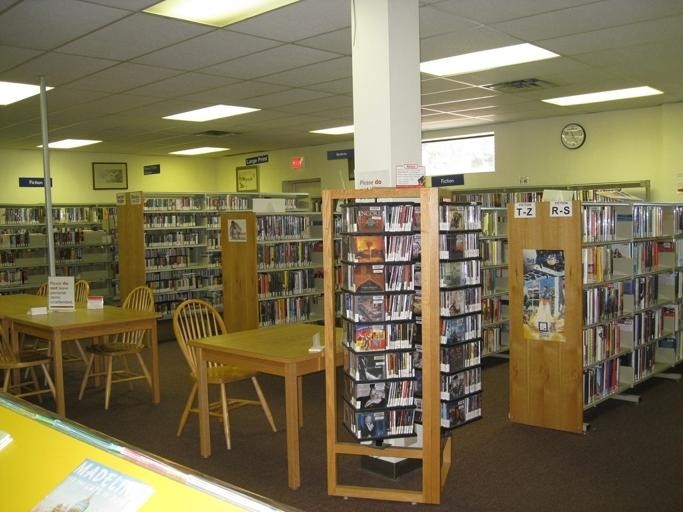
[561,123,586,150]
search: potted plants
[92,161,128,190]
[235,165,258,192]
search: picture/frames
[185,322,343,492]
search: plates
[452,179,651,358]
[506,199,683,434]
[336,202,482,444]
[0,205,118,305]
[221,210,325,334]
[116,192,254,322]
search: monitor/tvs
[30,459,152,507]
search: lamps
[0,280,163,417]
[173,299,277,450]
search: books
[257,199,322,326]
[332,205,422,444]
[575,189,620,203]
[674,206,683,360]
[0,208,120,296]
[30,459,152,507]
[580,205,621,405]
[453,192,543,208]
[439,205,482,428]
[479,211,509,356]
[632,205,664,380]
[144,197,248,314]
